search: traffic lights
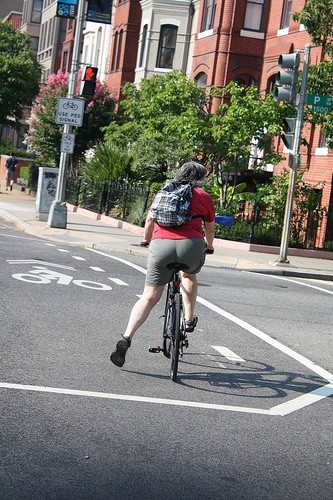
[273,46,301,157]
[79,66,98,97]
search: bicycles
[139,243,214,381]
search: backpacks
[148,178,202,228]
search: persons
[110,162,215,367]
[4,151,17,193]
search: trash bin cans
[36,166,59,223]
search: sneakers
[110,340,130,367]
[185,315,198,333]
